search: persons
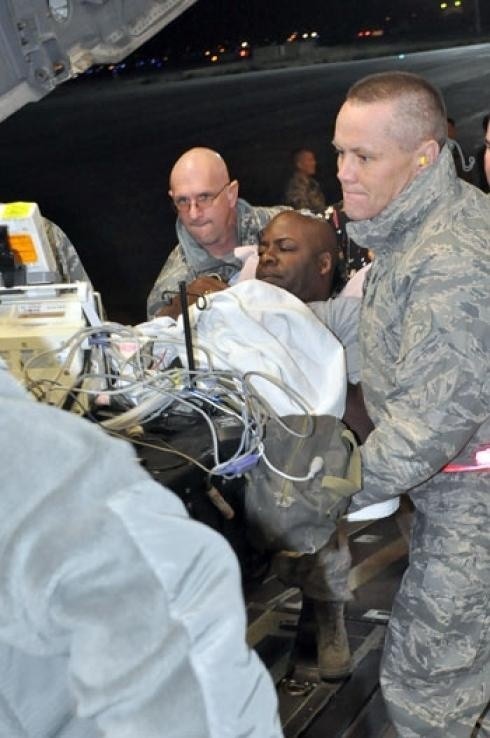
[331,68,489,738]
[0,358,285,737]
[285,144,330,213]
[143,146,355,685]
[447,116,473,184]
[151,204,376,446]
[479,112,489,188]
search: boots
[314,598,353,680]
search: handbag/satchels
[245,414,353,556]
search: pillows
[231,243,375,326]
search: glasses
[173,180,232,214]
